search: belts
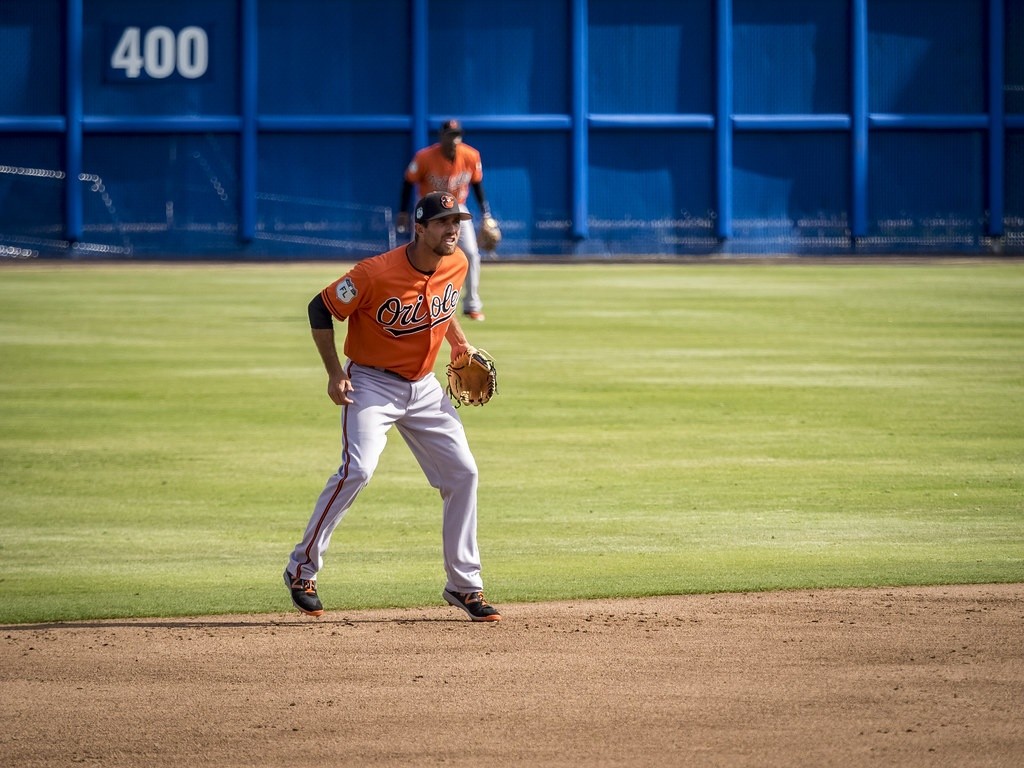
[368,365,409,382]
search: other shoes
[462,311,485,321]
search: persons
[396,120,501,321]
[283,191,501,621]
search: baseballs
[397,225,406,234]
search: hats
[415,191,473,222]
[441,120,463,134]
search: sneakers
[284,569,324,617]
[443,588,500,622]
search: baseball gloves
[477,216,501,251]
[445,349,497,407]
